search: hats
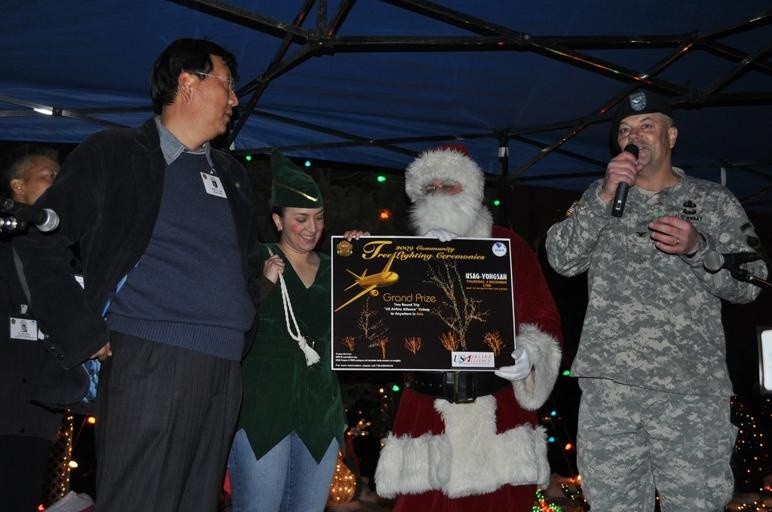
[271,145,324,211]
[615,90,673,123]
[404,144,485,233]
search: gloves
[424,229,457,242]
[495,347,532,382]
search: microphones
[611,143,640,217]
[2,199,61,233]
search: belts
[402,369,511,403]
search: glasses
[424,180,454,195]
[184,69,235,91]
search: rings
[673,237,680,247]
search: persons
[223,148,371,512]
[0,144,98,511]
[543,90,768,512]
[8,37,273,512]
[341,143,567,511]
